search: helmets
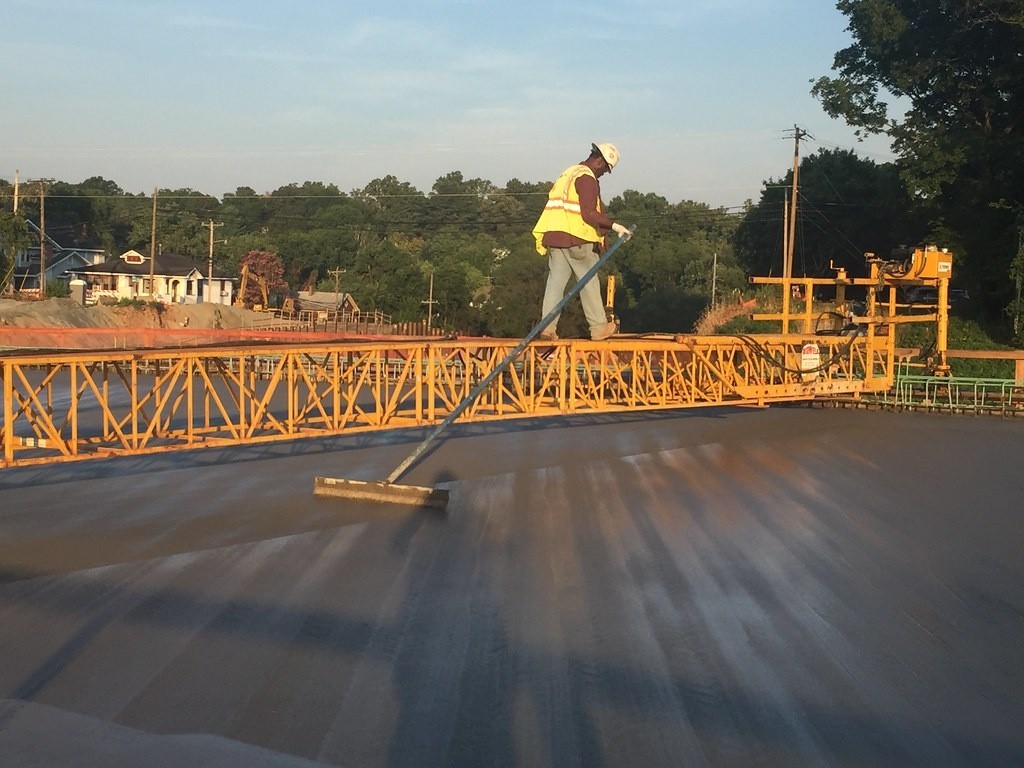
[592,142,620,174]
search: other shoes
[591,322,617,340]
[539,329,559,341]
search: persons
[532,143,632,341]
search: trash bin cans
[69,279,87,305]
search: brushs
[312,224,638,509]
